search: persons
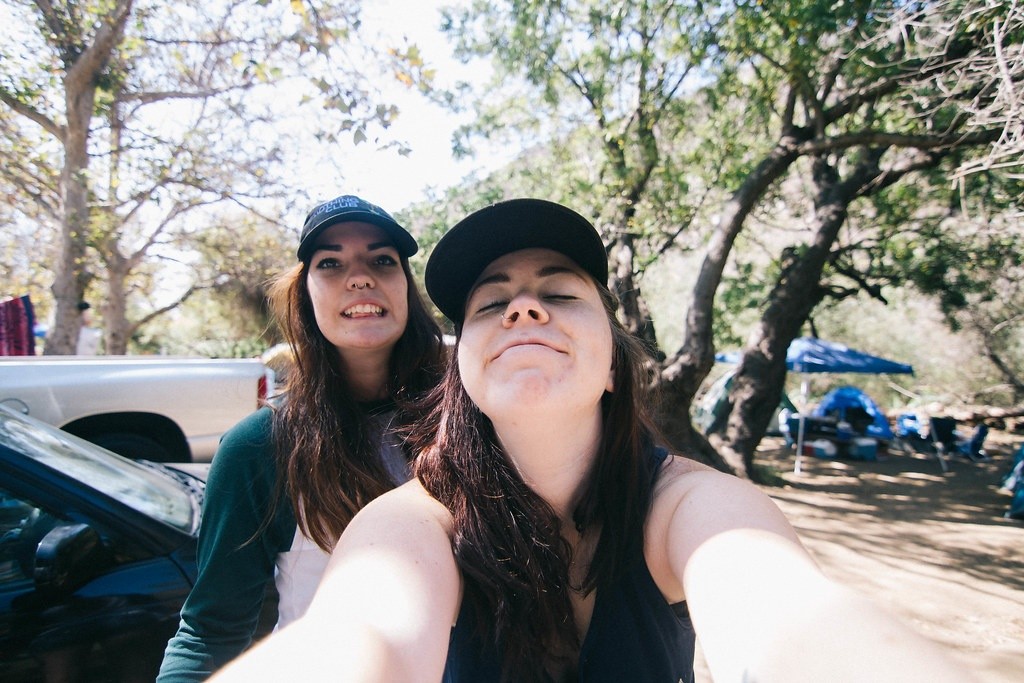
[206,198,886,683]
[154,197,454,683]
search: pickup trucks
[0,354,276,459]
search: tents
[701,364,804,438]
[810,384,896,444]
[786,336,949,473]
[996,444,1024,519]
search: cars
[0,399,282,681]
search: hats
[296,195,419,258]
[425,197,609,323]
